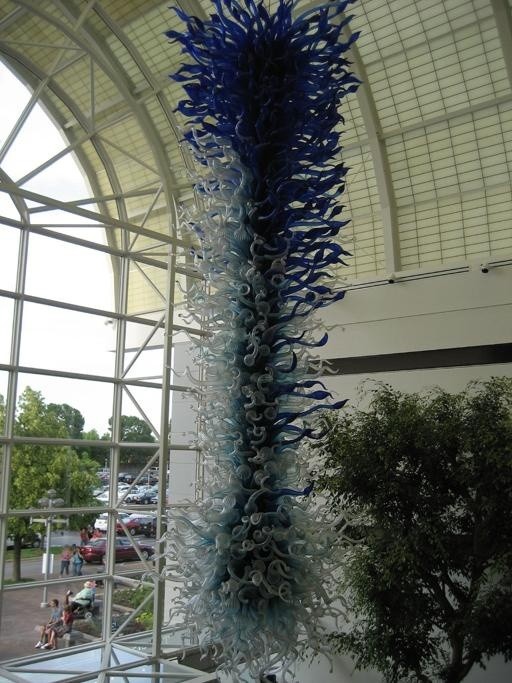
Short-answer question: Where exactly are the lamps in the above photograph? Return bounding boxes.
[479,259,490,273]
[385,271,397,283]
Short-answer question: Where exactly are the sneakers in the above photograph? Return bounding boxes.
[35,643,42,648]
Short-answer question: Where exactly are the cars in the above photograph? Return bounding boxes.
[81,536,155,564]
[90,465,170,503]
[6,529,41,550]
[92,511,166,538]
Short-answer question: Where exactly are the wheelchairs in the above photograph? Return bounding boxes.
[69,591,95,620]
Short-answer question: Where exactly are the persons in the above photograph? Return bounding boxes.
[94,528,102,538]
[40,606,73,650]
[87,527,94,538]
[72,547,83,576]
[70,581,94,612]
[59,544,71,578]
[80,527,89,545]
[35,599,63,648]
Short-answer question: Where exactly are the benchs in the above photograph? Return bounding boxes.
[33,621,84,650]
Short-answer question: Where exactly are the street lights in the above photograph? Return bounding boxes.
[28,489,70,608]
[105,458,108,477]
